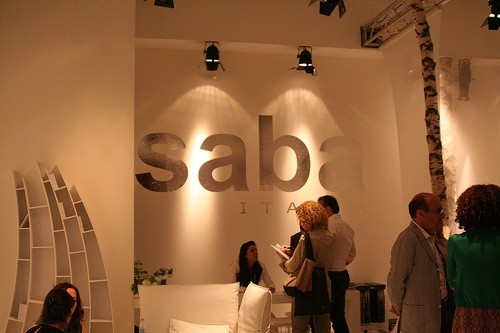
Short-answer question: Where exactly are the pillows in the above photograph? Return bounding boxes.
[238,281,272,333]
[138,282,240,333]
[170,318,229,333]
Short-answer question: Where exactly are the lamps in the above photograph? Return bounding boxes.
[480,0,500,30]
[194,41,225,72]
[288,46,317,76]
[308,0,346,18]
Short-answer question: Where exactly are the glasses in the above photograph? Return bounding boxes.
[422,208,444,214]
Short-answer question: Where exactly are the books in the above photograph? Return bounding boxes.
[271,242,291,260]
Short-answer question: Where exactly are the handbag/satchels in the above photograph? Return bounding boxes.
[283,232,317,297]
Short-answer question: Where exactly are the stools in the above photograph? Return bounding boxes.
[270,317,292,333]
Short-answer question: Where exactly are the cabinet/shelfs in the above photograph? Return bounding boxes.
[2,161,114,333]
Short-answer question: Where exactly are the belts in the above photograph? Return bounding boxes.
[441,296,450,308]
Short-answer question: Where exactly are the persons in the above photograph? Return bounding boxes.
[228,239,278,319]
[316,194,357,332]
[273,200,337,332]
[53,282,84,332]
[387,192,455,333]
[437,219,449,250]
[25,287,75,333]
[446,183,500,332]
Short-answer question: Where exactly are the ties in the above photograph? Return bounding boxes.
[434,240,453,309]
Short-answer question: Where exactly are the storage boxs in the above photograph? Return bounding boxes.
[354,282,387,326]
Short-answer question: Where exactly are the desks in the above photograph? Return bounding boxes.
[238,286,360,333]
